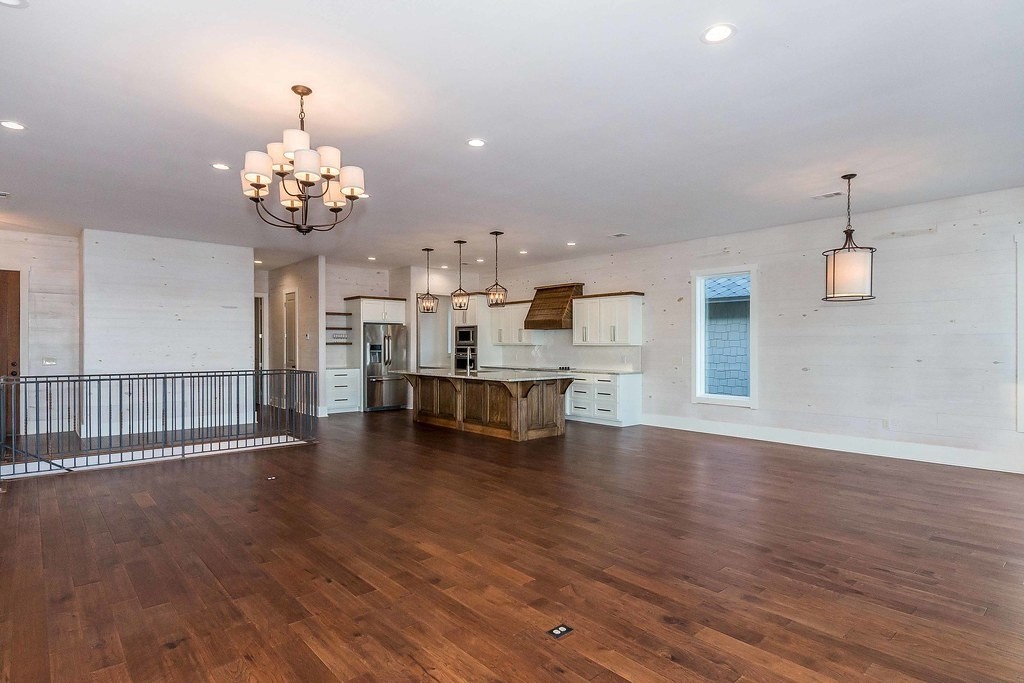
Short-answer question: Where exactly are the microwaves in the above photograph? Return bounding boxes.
[453,325,477,345]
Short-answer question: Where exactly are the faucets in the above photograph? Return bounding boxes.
[465,346,472,377]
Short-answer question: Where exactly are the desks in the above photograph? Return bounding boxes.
[390,365,575,444]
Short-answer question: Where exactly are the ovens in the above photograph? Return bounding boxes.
[455,346,477,378]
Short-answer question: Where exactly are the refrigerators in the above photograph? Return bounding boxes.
[363,322,406,412]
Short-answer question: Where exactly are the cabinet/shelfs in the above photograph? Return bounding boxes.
[325,369,362,413]
[571,368,640,423]
[570,292,644,346]
[491,303,545,346]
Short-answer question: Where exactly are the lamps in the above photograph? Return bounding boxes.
[240,84,368,236]
[820,172,879,304]
[418,248,439,315]
[450,238,471,312]
[486,231,508,309]
